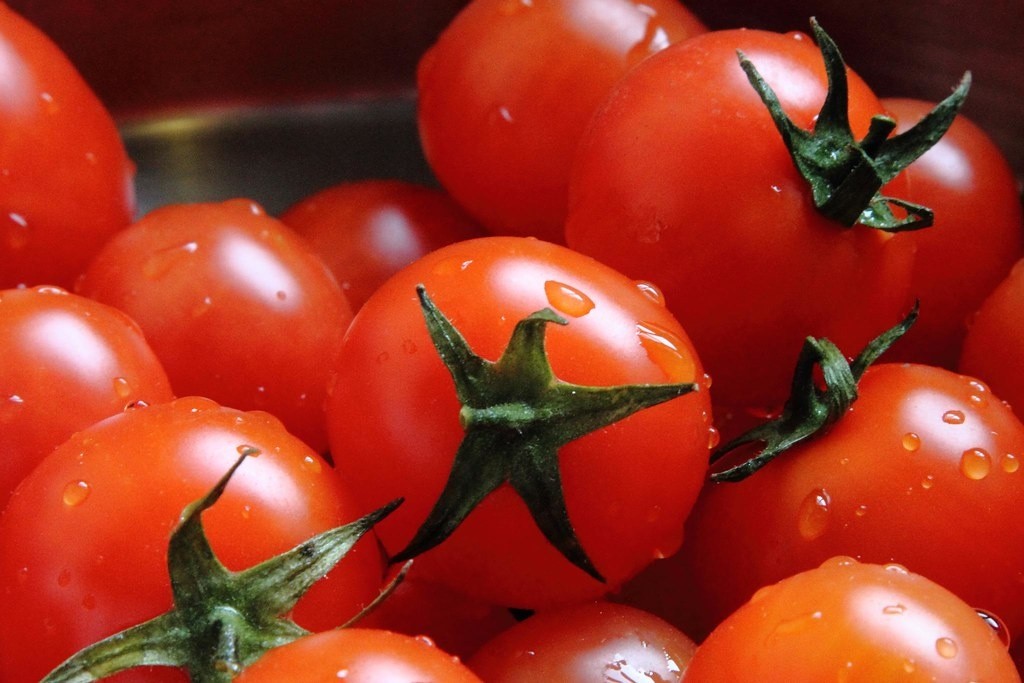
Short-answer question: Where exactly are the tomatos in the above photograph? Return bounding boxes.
[0,0,1024,682]
